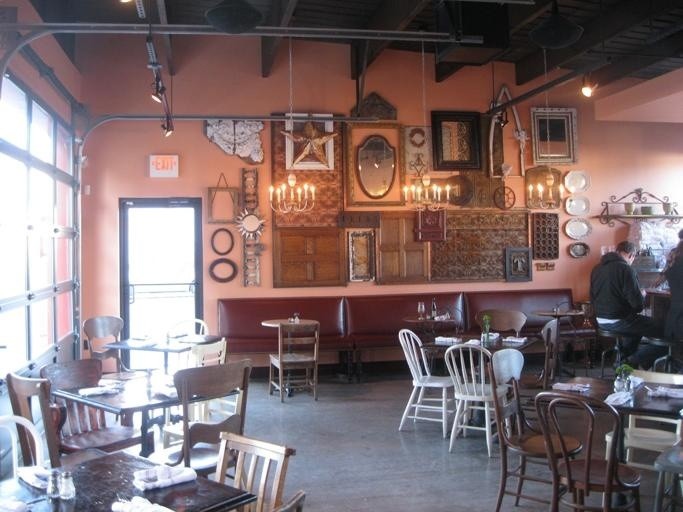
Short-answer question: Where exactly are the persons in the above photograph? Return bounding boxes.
[650,229,683,289]
[589,241,669,371]
[651,241,683,374]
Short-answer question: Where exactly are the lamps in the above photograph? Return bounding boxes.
[152,69,167,104]
[161,104,175,138]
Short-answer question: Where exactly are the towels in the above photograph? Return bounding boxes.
[464,338,481,346]
[628,374,645,389]
[603,391,633,406]
[97,378,125,389]
[434,335,462,343]
[482,332,500,340]
[552,382,591,393]
[130,464,198,491]
[1,496,27,512]
[130,334,147,342]
[15,465,52,490]
[154,382,180,399]
[109,496,177,512]
[656,386,682,399]
[501,335,528,344]
[77,386,118,398]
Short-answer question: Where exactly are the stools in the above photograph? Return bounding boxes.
[640,335,683,373]
[597,330,636,379]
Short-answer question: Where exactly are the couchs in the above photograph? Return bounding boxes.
[209,287,595,377]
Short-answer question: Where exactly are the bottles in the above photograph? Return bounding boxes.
[59,472,76,500]
[432,297,437,320]
[46,471,60,499]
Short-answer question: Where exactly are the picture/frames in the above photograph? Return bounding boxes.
[285,111,334,171]
[530,106,580,167]
[430,110,483,171]
[345,119,405,209]
[504,245,533,283]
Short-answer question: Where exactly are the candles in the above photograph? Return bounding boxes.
[437,187,441,201]
[269,186,274,204]
[304,184,309,201]
[276,188,281,206]
[540,187,544,200]
[537,184,540,197]
[403,187,408,203]
[445,183,451,200]
[411,185,415,199]
[558,185,565,202]
[528,184,531,199]
[433,185,438,200]
[297,189,302,202]
[311,186,316,202]
[280,184,286,201]
[416,187,422,201]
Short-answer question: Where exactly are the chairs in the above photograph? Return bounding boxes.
[605,369,683,512]
[490,376,584,512]
[534,389,645,511]
[190,338,229,368]
[146,358,253,477]
[520,319,559,435]
[2,415,45,477]
[475,307,528,349]
[82,315,150,382]
[3,371,105,466]
[216,431,307,510]
[38,358,155,459]
[444,343,512,456]
[398,329,454,439]
[475,308,527,338]
[194,319,206,336]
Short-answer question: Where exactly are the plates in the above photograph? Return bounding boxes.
[565,218,592,240]
[565,170,591,194]
[570,242,590,258]
[565,195,591,217]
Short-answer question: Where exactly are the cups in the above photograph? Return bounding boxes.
[417,302,425,321]
